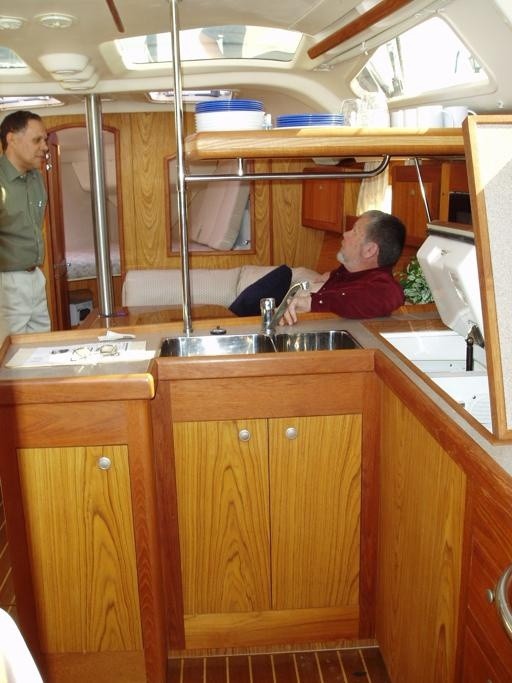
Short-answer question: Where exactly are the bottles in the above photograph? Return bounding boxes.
[343,92,390,130]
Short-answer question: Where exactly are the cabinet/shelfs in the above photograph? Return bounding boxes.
[391,163,449,247]
[373,352,512,683]
[150,373,376,653]
[3,393,169,683]
[301,164,364,235]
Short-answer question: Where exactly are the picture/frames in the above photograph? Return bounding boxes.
[164,155,256,256]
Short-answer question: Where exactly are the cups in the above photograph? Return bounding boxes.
[392,107,477,127]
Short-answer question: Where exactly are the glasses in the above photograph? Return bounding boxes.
[70,344,118,362]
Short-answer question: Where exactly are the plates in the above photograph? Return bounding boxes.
[193,102,346,131]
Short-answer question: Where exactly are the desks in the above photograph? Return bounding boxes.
[79,302,235,330]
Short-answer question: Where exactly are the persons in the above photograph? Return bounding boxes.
[0,110,51,347]
[274,210,405,326]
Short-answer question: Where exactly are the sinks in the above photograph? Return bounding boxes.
[273,332,362,349]
[157,335,274,354]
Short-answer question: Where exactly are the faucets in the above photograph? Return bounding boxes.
[258,280,310,330]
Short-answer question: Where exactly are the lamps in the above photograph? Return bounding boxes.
[50,67,94,81]
[59,74,99,90]
[38,53,89,74]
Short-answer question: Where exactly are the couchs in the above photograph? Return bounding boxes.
[121,264,326,315]
